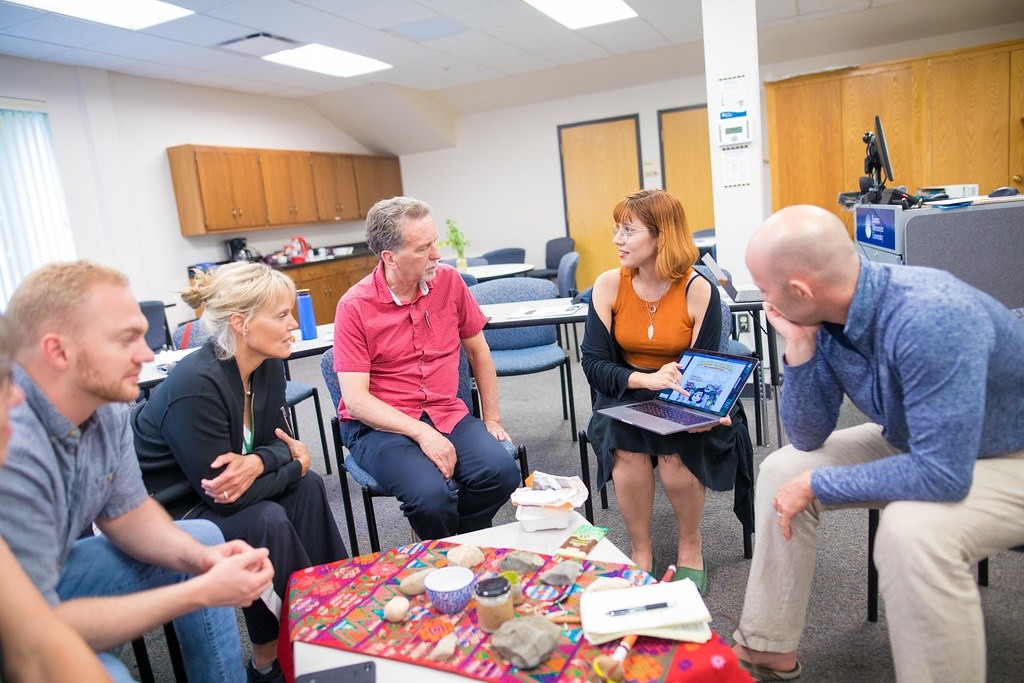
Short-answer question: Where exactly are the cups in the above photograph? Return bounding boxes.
[317,248,328,258]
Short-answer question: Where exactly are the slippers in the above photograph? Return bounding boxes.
[644,556,656,578]
[672,562,707,596]
[732,647,801,679]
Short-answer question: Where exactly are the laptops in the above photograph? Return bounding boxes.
[701,252,765,302]
[597,346,759,436]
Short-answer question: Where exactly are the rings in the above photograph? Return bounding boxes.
[223,491,229,500]
[674,379,677,384]
[777,512,782,517]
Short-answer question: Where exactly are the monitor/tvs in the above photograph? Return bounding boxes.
[864,115,894,203]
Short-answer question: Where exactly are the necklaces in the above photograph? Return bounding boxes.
[244,381,252,396]
[637,273,668,340]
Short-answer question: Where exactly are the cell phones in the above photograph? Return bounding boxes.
[296,662,376,683]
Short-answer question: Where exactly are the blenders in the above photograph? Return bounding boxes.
[225,237,253,262]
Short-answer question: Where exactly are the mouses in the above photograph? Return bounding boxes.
[930,193,949,200]
[988,187,1017,197]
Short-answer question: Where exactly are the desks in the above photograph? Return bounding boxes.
[480,296,589,330]
[466,264,533,280]
[138,322,336,388]
[276,512,753,683]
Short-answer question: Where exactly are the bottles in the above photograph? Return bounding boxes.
[296,293,318,339]
[500,570,524,604]
[474,577,514,634]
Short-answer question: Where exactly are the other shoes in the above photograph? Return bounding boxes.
[247,657,286,683]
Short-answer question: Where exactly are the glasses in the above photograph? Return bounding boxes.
[610,225,655,241]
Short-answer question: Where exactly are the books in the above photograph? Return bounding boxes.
[580,577,713,644]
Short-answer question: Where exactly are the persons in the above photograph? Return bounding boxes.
[130,261,352,683]
[580,189,732,597]
[333,196,521,542]
[0,258,274,683]
[731,204,1023,682]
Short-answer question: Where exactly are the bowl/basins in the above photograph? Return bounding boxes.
[333,247,354,256]
[424,566,474,614]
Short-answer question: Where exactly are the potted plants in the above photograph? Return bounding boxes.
[437,218,470,271]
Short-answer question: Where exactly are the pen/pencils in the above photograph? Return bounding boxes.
[425,310,430,327]
[608,602,668,616]
[553,616,580,623]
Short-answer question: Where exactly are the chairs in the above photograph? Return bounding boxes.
[137,301,168,352]
[468,277,578,442]
[321,342,529,558]
[173,317,333,475]
[483,248,526,264]
[580,301,753,559]
[526,237,575,279]
[556,251,582,363]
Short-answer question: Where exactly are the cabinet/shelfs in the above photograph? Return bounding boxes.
[167,145,404,237]
[283,254,381,328]
